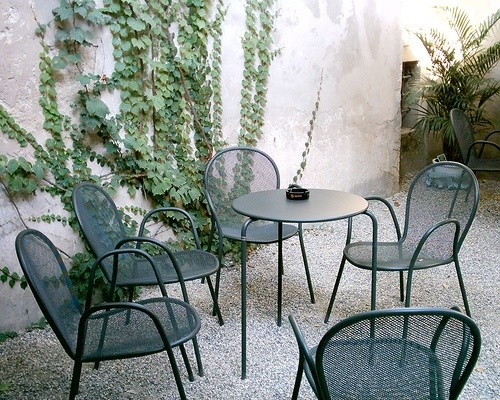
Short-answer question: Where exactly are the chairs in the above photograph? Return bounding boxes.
[201,146,315,316]
[288,305,481,400]
[72,183,224,377]
[447,108,500,219]
[324,161,480,336]
[15,229,195,400]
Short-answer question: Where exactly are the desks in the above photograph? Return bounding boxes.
[232,189,378,380]
